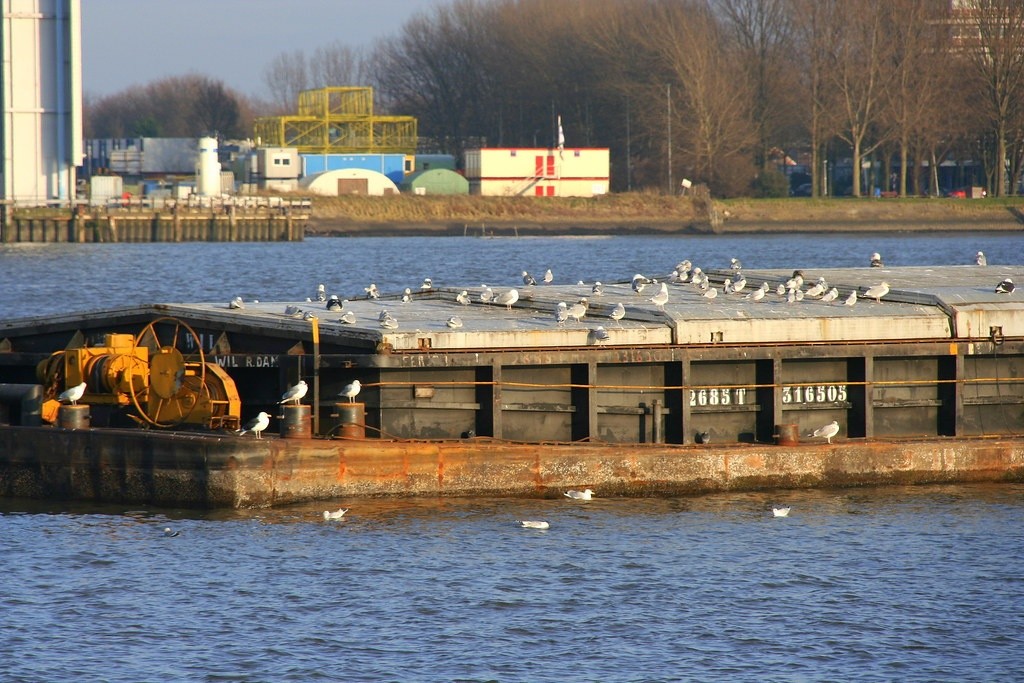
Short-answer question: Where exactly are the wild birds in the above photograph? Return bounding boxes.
[339,278,519,329]
[976,252,986,265]
[254,284,343,311]
[276,381,308,405]
[995,279,1015,293]
[862,282,890,303]
[870,253,883,268]
[240,412,272,439]
[515,489,790,529]
[337,380,362,404]
[521,258,857,348]
[165,509,348,538]
[230,297,244,309]
[808,421,839,444]
[285,305,318,322]
[58,381,88,406]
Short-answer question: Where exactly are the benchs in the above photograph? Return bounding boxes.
[881,192,899,198]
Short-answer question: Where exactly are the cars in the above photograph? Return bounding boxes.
[777,164,985,198]
